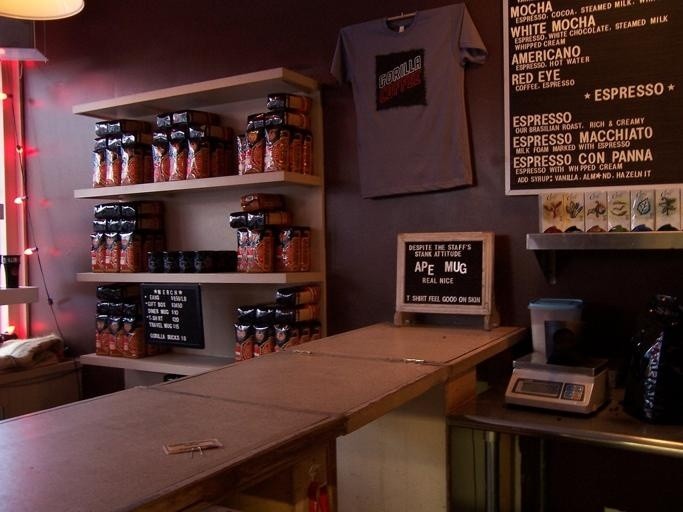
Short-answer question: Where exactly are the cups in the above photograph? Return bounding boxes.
[2,254,20,288]
[543,319,566,364]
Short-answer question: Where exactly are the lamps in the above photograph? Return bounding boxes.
[0,0,84,21]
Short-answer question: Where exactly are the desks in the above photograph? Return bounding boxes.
[465,360,683,509]
[0,323,531,510]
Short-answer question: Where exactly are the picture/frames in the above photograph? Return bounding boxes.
[393,231,499,333]
[499,0,682,197]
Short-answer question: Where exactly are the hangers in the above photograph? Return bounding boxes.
[385,7,416,33]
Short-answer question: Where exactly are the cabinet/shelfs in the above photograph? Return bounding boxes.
[72,67,328,392]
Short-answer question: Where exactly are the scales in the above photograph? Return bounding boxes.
[504,352,610,415]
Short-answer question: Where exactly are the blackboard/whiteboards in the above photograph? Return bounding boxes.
[395,232,495,316]
[140,282,206,349]
